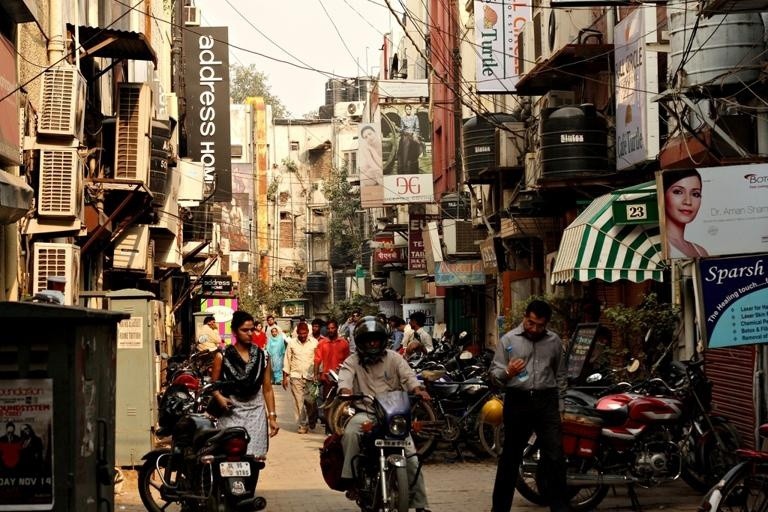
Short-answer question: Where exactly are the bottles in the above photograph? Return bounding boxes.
[506,345,531,384]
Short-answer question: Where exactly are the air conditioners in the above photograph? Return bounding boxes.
[441,218,490,257]
[280,301,305,318]
[27,64,219,311]
[345,101,367,118]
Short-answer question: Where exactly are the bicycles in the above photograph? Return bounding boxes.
[646,319,691,379]
[697,422,768,511]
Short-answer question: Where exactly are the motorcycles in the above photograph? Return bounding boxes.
[167,334,223,412]
[514,354,645,508]
[138,378,267,512]
[315,330,504,460]
[333,391,434,511]
[534,338,751,512]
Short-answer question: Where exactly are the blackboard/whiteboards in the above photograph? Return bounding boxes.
[567,323,601,378]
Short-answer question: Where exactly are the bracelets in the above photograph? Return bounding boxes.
[269,412,277,418]
[505,369,511,377]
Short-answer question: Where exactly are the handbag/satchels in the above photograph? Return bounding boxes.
[319,432,346,492]
[206,348,232,419]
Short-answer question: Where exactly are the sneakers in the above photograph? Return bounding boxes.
[297,424,308,433]
[309,416,316,429]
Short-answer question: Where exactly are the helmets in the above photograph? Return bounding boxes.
[353,315,389,357]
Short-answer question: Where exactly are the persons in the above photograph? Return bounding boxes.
[359,126,382,168]
[663,170,710,257]
[488,301,567,511]
[334,314,433,512]
[227,196,249,251]
[206,309,280,500]
[19,424,43,476]
[197,316,222,354]
[252,310,433,437]
[392,104,420,144]
[0,422,20,477]
[232,166,246,194]
